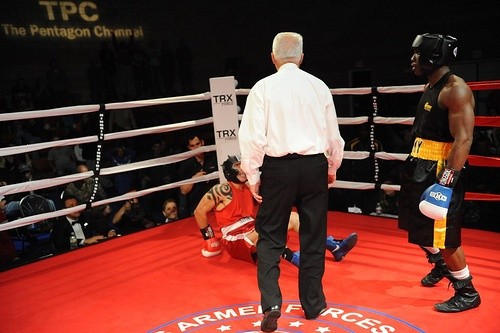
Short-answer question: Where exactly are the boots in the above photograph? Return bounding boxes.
[434,274,481,312]
[420,252,447,286]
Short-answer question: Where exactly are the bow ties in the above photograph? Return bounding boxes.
[72,220,80,225]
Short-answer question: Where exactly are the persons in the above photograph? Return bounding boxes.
[397,34,481,313]
[236,32,345,332]
[329,89,500,231]
[0,30,218,270]
[194,155,358,269]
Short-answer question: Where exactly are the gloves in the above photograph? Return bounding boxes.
[200,224,222,257]
[418,166,460,221]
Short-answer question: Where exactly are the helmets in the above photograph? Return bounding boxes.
[413,32,459,74]
[221,154,245,184]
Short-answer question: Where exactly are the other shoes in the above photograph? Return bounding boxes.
[261,305,281,332]
[305,301,327,319]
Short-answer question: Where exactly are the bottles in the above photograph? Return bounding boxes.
[375,202,382,217]
[69,232,78,250]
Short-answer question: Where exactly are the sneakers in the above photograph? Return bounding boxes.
[326,233,357,261]
[292,250,300,266]
